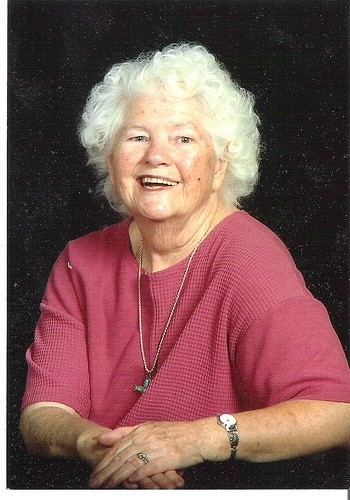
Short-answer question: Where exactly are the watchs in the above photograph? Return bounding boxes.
[216,413,241,462]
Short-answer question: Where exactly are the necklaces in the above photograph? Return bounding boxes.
[133,208,227,392]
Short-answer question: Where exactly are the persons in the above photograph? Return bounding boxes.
[19,41,349,488]
